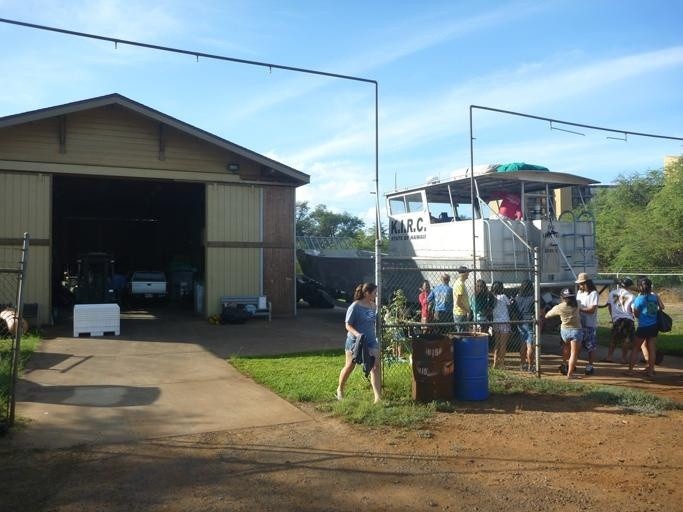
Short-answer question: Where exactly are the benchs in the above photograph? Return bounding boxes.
[220,295,272,322]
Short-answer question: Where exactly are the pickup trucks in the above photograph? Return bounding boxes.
[127,270,170,306]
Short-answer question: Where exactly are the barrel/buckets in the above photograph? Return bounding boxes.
[412,333,454,401]
[0,307,30,334]
[449,333,489,400]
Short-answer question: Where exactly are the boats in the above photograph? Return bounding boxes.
[293,165,602,333]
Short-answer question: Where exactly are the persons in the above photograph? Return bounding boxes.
[384,294,409,362]
[470,279,497,367]
[512,280,546,374]
[418,280,432,334]
[453,266,472,332]
[491,281,513,370]
[575,273,599,375]
[336,283,383,403]
[544,288,584,379]
[600,278,665,377]
[426,273,456,335]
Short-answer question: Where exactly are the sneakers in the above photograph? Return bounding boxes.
[656,349,663,364]
[585,364,594,373]
[558,362,576,374]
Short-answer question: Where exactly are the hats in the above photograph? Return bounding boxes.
[561,288,575,298]
[575,273,591,284]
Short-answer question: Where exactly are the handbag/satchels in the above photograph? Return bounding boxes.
[658,310,672,333]
